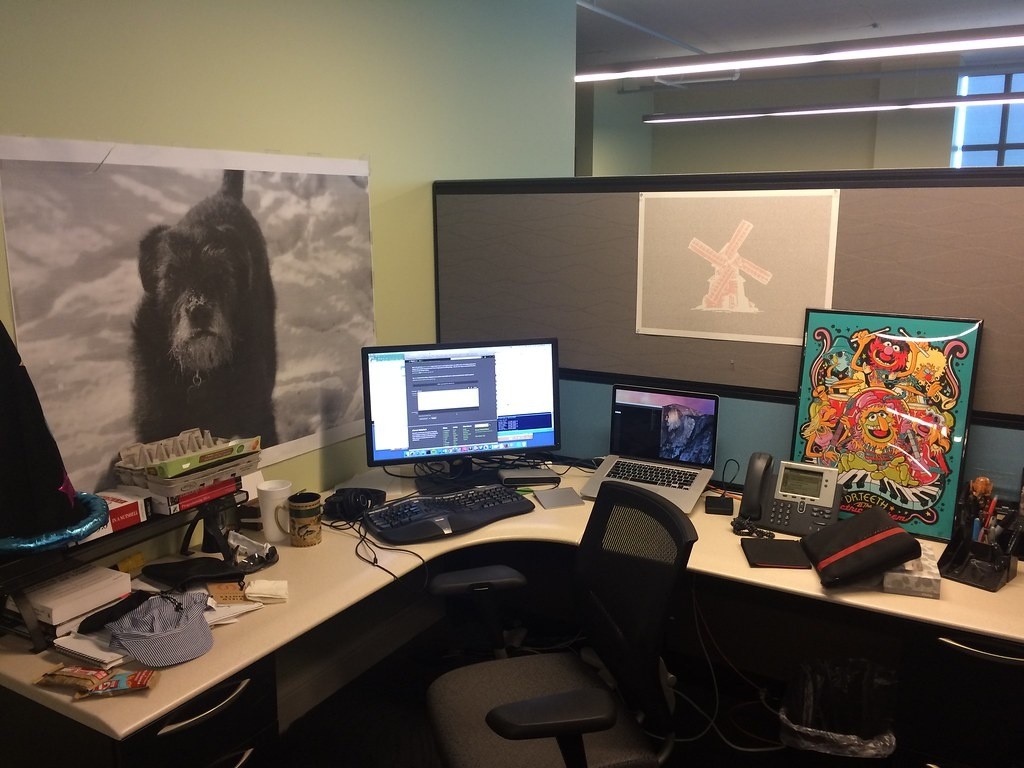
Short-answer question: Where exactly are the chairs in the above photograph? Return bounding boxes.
[424,480,697,768]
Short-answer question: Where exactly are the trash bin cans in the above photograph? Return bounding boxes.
[773,660,900,768]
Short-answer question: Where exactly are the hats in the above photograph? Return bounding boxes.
[102,589,214,666]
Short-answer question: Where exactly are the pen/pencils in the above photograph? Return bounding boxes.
[972,494,1023,550]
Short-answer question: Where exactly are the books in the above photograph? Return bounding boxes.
[42,487,153,548]
[3,565,265,670]
[116,475,242,514]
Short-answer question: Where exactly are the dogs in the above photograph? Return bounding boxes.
[125,168,281,452]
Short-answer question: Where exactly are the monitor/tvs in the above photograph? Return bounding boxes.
[361,337,561,496]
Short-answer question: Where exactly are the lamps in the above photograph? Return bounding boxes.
[575,24,1024,86]
[642,91,1024,124]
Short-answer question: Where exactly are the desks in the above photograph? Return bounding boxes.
[0,453,1024,768]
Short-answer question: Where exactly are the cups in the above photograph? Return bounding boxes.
[274,492,323,547]
[255,480,293,542]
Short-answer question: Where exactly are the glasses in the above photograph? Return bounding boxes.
[235,546,279,574]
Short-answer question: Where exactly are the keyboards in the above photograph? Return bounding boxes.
[362,485,535,545]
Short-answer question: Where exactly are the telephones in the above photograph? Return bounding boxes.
[735,451,846,537]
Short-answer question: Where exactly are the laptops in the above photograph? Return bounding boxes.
[580,384,720,514]
